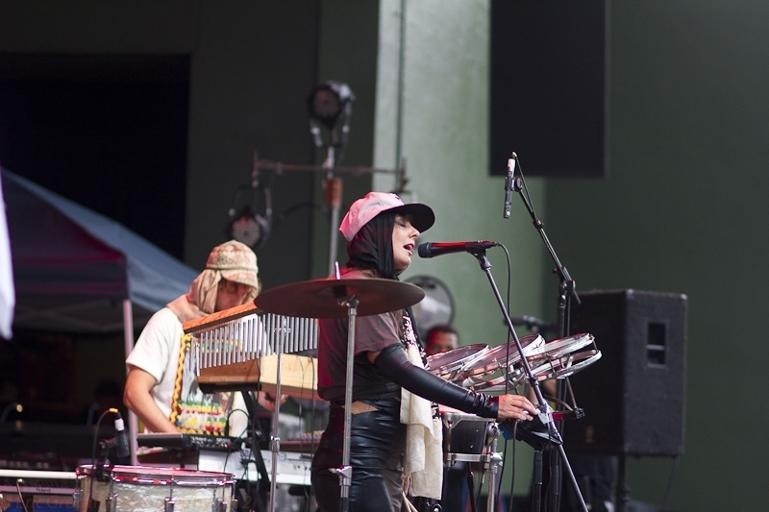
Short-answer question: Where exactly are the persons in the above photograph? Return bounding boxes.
[310,192,537,511]
[86,379,126,419]
[403,322,496,510]
[126,235,287,461]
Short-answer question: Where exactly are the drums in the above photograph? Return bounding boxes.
[462,335,544,390]
[525,333,602,381]
[75,465,238,512]
[442,411,497,462]
[425,343,498,382]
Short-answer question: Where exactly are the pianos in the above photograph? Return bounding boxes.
[101,431,323,486]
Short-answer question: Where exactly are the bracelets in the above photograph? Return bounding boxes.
[263,392,284,404]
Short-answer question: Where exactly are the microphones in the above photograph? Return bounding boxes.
[417,238,498,258]
[503,158,516,218]
[112,412,131,465]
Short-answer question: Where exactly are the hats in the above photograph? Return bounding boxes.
[203,238,261,292]
[338,190,436,243]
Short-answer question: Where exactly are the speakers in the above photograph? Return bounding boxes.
[562,288,691,458]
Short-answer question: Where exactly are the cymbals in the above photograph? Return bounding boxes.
[253,279,425,319]
